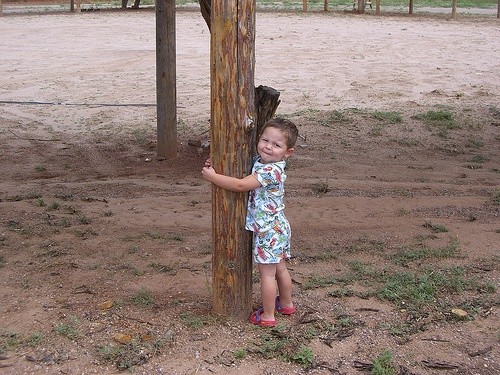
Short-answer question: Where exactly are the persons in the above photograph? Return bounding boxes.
[201,116,295,328]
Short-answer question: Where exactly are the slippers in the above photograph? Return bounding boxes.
[249,307,275,327]
[275,295,295,315]
